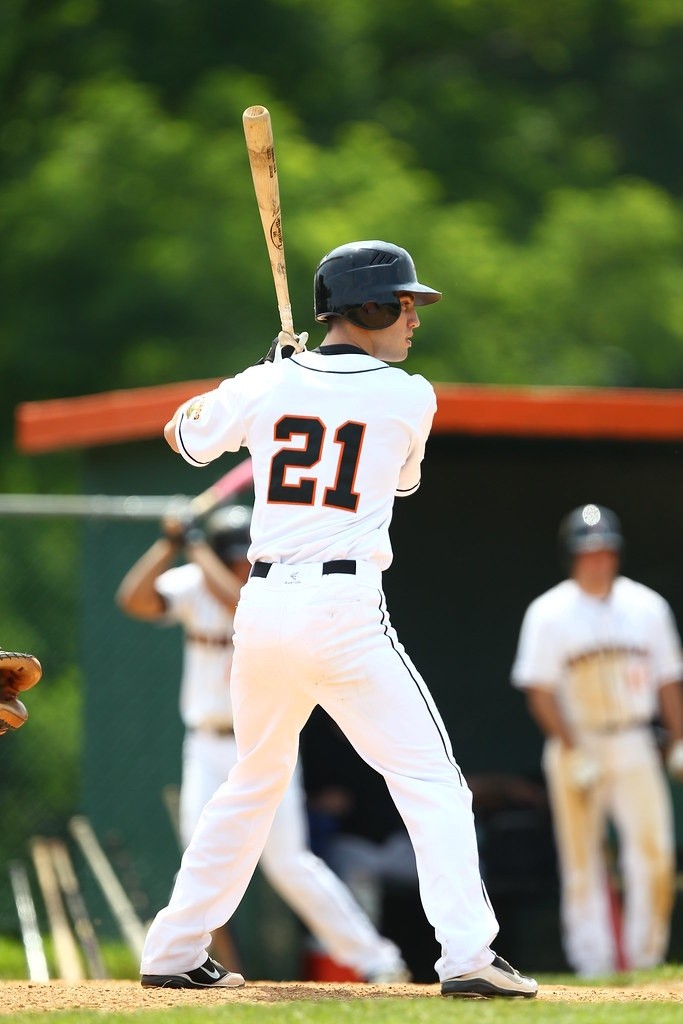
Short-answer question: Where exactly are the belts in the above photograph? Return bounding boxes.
[183,725,236,739]
[599,716,653,737]
[250,560,358,579]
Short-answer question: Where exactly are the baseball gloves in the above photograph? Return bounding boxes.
[0,645,43,735]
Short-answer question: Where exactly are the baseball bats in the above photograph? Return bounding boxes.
[11,810,149,980]
[176,458,252,519]
[241,104,297,342]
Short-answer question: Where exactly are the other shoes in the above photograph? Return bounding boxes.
[575,965,621,983]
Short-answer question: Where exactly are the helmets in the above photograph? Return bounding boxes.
[558,502,625,567]
[202,504,254,567]
[313,239,443,331]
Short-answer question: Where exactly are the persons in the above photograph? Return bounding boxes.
[118,504,413,984]
[140,240,538,1000]
[510,505,683,975]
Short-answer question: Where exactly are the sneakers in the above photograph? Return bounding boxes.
[440,953,540,1003]
[367,969,411,985]
[140,953,246,990]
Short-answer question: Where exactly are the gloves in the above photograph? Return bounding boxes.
[561,736,598,802]
[253,330,309,365]
[665,737,683,784]
[162,496,208,546]
